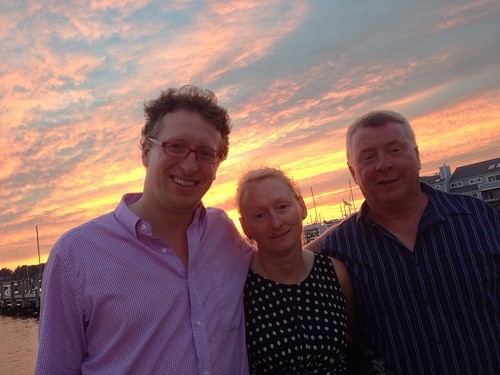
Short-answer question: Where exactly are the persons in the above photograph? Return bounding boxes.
[233,167,372,375]
[319,112,500,375]
[34,84,340,375]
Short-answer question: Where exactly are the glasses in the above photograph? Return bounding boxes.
[147,135,223,164]
[245,202,300,225]
